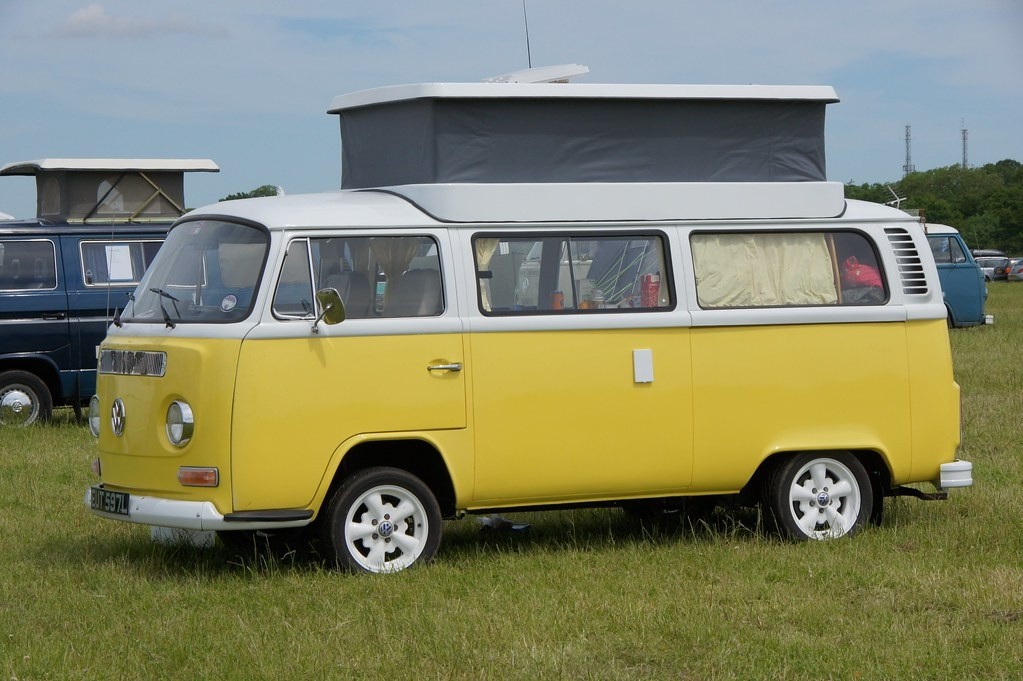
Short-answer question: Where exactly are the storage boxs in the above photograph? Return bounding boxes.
[985,315,993,324]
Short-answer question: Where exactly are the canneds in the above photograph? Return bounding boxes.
[551,290,564,309]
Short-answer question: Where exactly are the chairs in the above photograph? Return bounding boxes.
[382,268,442,316]
[341,269,372,318]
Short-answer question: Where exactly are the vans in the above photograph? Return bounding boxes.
[586,222,992,329]
[0,157,330,434]
[79,79,977,575]
[971,257,1010,283]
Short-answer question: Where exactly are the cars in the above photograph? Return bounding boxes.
[1006,258,1023,281]
[993,257,1022,280]
